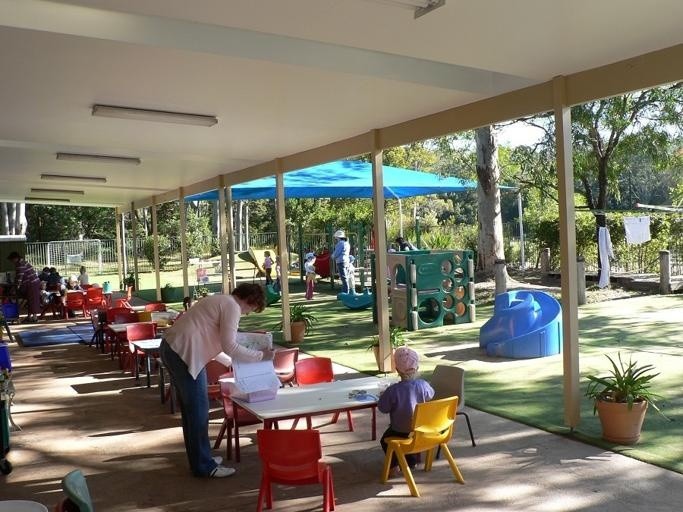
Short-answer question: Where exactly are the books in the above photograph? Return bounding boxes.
[233,331,281,395]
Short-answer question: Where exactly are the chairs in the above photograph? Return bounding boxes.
[1,281,111,320]
[429,366,476,461]
[290,356,355,435]
[213,372,279,463]
[256,429,335,512]
[90,298,302,420]
[381,396,465,497]
[1,320,96,510]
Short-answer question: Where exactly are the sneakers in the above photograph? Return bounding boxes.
[210,456,224,465]
[200,466,237,479]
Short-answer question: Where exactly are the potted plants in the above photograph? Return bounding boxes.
[583,351,667,445]
[367,327,410,372]
[272,303,318,343]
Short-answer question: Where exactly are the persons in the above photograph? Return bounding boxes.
[169,295,192,325]
[330,230,350,300]
[303,252,317,300]
[261,250,273,285]
[347,255,356,294]
[6,251,41,324]
[394,236,419,251]
[274,255,282,292]
[196,264,208,284]
[37,266,87,317]
[385,249,395,279]
[376,346,434,476]
[158,283,277,477]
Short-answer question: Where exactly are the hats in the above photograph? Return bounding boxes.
[333,230,345,238]
[395,348,419,374]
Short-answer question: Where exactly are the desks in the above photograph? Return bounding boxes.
[229,377,392,462]
